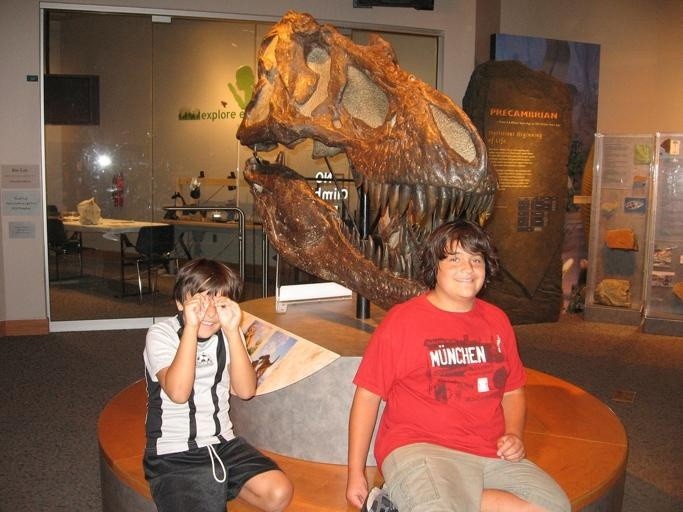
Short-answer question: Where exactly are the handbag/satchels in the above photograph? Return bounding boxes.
[606,229,638,251]
[593,278,631,307]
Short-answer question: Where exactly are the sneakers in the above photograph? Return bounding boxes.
[360,481,399,512]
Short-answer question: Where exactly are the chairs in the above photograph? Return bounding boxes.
[47,218,83,281]
[120,225,175,293]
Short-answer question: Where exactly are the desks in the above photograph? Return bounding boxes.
[63,219,169,293]
[160,219,262,260]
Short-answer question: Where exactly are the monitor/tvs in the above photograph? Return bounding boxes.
[45,74,100,126]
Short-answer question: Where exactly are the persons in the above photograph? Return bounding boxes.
[142,259,293,512]
[346,220,572,512]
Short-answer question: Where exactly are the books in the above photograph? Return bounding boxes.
[181,215,210,222]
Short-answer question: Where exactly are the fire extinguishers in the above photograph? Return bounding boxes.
[112,172,124,208]
